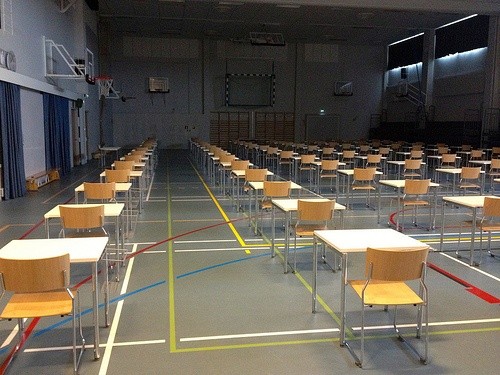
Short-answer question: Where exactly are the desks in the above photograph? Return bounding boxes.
[0,236,110,360]
[100,170,145,217]
[310,161,346,195]
[219,159,253,197]
[395,151,424,159]
[425,147,450,154]
[333,152,358,162]
[353,155,386,169]
[457,150,486,162]
[313,227,430,346]
[112,162,146,169]
[44,203,125,280]
[371,148,393,154]
[291,156,319,184]
[232,169,273,213]
[426,155,461,169]
[270,197,347,273]
[77,182,133,239]
[378,180,440,232]
[387,159,427,180]
[336,169,383,208]
[469,160,493,172]
[434,167,486,196]
[247,181,301,236]
[439,195,500,266]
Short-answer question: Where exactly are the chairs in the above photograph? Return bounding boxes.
[0,137,161,375]
[190,134,500,367]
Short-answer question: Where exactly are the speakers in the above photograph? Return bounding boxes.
[401,68,408,79]
